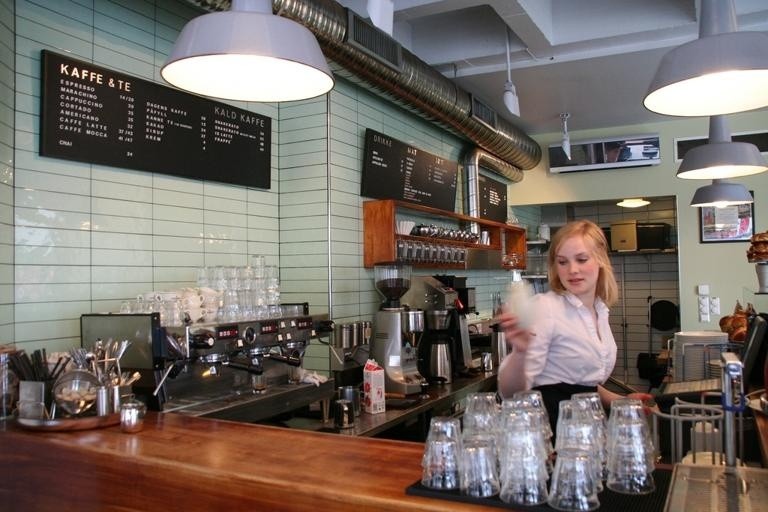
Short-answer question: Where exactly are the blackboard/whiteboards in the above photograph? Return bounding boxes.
[360,127,459,213]
[38,49,272,193]
[478,173,507,224]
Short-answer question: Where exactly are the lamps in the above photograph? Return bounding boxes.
[690,179,754,210]
[559,112,573,159]
[640,0,768,119]
[365,0,395,37]
[501,25,523,118]
[675,113,768,182]
[160,0,334,104]
[615,197,651,208]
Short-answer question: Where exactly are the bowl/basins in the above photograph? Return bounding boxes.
[394,220,415,236]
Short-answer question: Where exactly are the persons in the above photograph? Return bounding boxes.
[497,219,653,448]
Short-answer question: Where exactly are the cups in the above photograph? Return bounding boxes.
[420,389,664,511]
[200,254,282,326]
[334,400,356,429]
[96,386,120,418]
[119,384,133,406]
[118,400,147,434]
[120,287,207,326]
[480,352,493,370]
[502,251,519,267]
[18,380,44,418]
[535,233,541,241]
[338,386,367,417]
[481,231,489,245]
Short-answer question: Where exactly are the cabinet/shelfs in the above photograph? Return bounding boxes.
[363,200,528,270]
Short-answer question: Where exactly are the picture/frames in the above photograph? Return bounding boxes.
[698,190,755,244]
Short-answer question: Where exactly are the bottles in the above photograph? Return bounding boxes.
[334,321,372,347]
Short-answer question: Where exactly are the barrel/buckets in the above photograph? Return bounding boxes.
[650,328,676,355]
[610,219,637,251]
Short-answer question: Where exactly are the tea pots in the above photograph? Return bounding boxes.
[413,224,479,243]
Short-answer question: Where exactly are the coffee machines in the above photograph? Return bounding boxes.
[80,302,337,422]
[400,276,459,384]
[433,274,479,375]
[373,264,429,394]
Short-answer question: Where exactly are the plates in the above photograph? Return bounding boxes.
[672,331,730,382]
[15,413,122,431]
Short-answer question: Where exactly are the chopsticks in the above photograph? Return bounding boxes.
[7,348,72,407]
